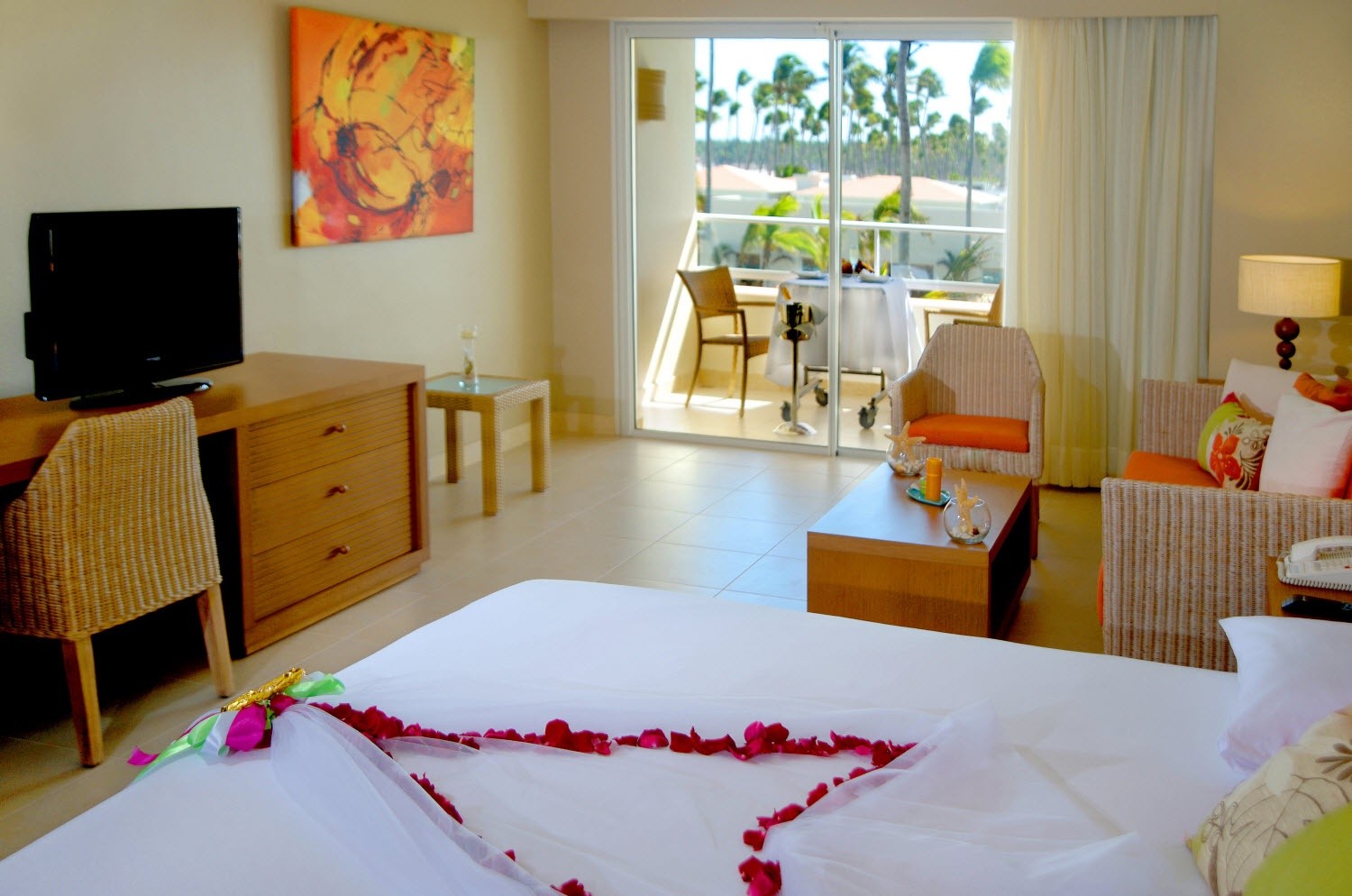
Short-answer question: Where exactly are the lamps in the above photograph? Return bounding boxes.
[1235,255,1342,369]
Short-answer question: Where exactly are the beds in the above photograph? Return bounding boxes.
[0,579,1351,896]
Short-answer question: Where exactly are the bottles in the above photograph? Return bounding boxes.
[943,496,991,545]
[456,323,479,392]
[886,440,925,475]
[925,456,943,501]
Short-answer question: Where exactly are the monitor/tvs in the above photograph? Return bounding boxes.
[24,206,244,410]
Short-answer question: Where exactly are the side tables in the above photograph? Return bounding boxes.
[1262,556,1352,621]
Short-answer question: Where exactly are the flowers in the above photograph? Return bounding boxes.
[461,324,478,378]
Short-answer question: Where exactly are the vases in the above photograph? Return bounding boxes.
[459,339,480,388]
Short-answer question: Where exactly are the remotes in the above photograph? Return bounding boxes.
[1280,593,1352,623]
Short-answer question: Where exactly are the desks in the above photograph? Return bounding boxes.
[425,373,551,515]
[764,274,917,431]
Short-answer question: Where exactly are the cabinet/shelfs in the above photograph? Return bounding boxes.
[0,351,433,654]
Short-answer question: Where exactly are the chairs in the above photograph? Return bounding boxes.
[0,395,233,765]
[676,266,808,418]
[1098,374,1352,673]
[923,280,1003,344]
[890,323,1046,477]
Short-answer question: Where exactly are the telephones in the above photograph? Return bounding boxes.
[1276,534,1352,592]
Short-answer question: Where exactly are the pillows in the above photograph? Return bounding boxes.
[1196,392,1273,490]
[1218,615,1351,777]
[1258,372,1352,499]
[1184,703,1352,895]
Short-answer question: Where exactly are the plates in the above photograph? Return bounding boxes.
[905,484,951,507]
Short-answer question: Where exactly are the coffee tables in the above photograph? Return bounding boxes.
[807,460,1032,639]
